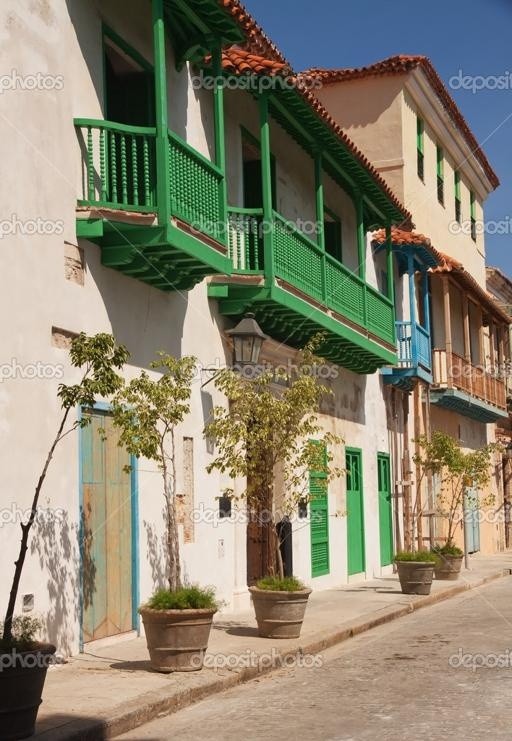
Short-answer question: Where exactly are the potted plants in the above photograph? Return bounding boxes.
[393,426,510,598]
[0,327,352,741]
